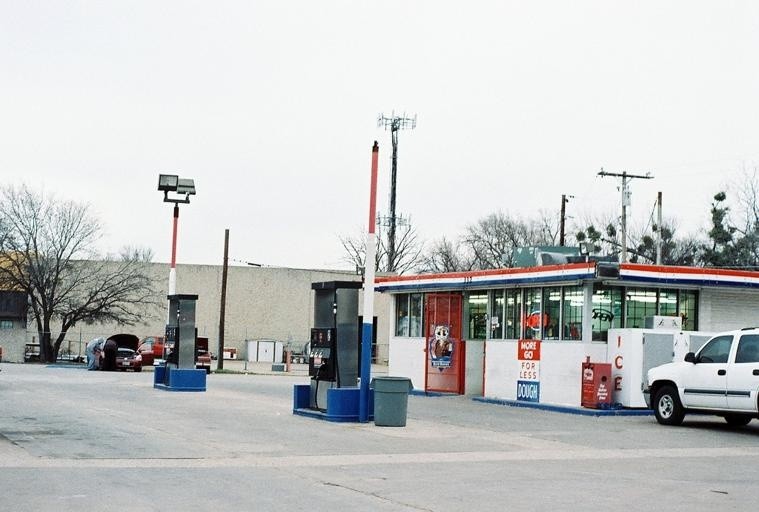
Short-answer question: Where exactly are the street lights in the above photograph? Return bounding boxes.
[157,174,196,365]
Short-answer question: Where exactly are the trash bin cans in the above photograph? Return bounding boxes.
[374,376,410,428]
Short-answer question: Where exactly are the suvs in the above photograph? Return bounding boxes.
[642,326,759,426]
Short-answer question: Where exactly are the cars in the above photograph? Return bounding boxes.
[166,337,212,373]
[138,335,165,360]
[93,333,142,372]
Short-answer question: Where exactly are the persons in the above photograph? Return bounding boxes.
[85,337,104,372]
[101,338,119,371]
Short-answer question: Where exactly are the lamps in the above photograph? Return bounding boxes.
[596,261,619,279]
[580,243,594,263]
